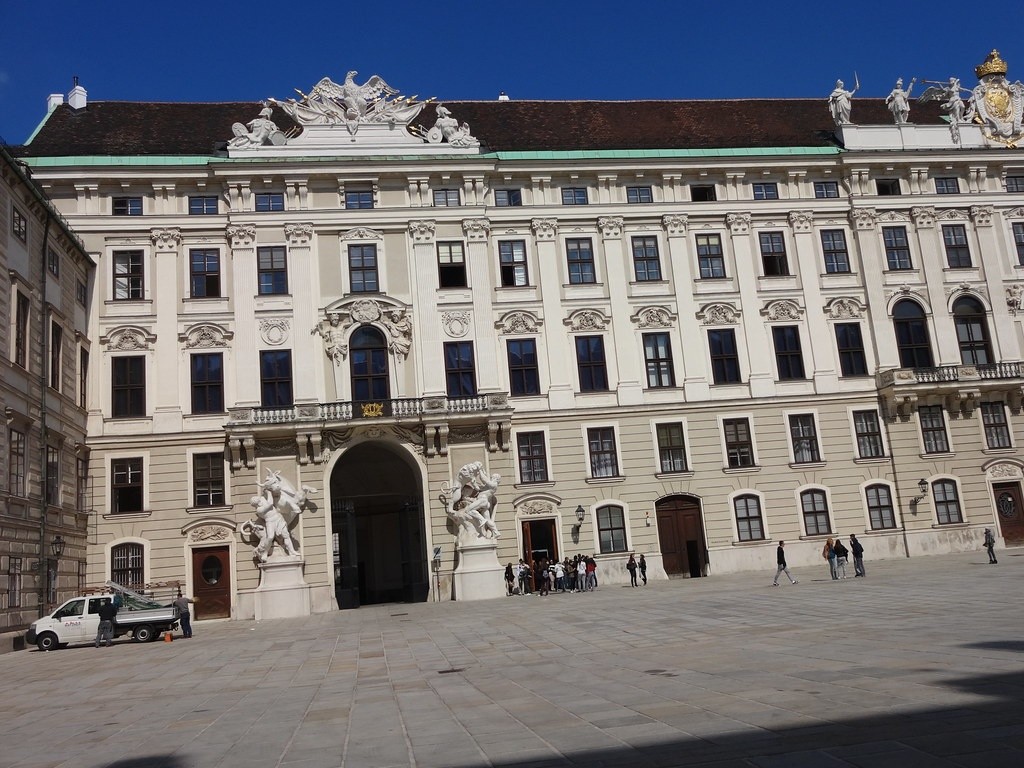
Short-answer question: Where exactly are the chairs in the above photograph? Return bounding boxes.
[89,600,98,614]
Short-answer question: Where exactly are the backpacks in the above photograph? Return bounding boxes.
[578,562,585,574]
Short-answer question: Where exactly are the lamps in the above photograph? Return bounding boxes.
[914,479,929,505]
[574,504,586,531]
[51,536,66,560]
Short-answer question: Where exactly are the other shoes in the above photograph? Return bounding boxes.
[793,581,800,584]
[773,583,779,586]
[538,594,542,596]
[635,585,639,587]
[96,645,99,648]
[992,560,997,563]
[106,643,113,647]
[545,594,548,596]
[989,560,993,564]
[525,592,531,595]
[183,636,191,638]
[632,585,634,588]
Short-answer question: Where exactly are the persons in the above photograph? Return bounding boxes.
[638,555,647,585]
[518,554,597,597]
[628,554,638,587]
[983,528,997,564]
[886,78,916,124]
[850,534,866,577]
[250,489,301,563]
[465,465,501,540]
[828,80,859,126]
[175,593,192,638]
[938,78,965,122]
[378,307,411,355]
[834,539,848,580]
[825,539,838,580]
[773,541,799,586]
[1008,284,1024,315]
[96,598,117,648]
[434,103,476,145]
[322,309,353,355]
[505,563,515,596]
[228,107,277,147]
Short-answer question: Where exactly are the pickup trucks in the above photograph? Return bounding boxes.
[25,592,180,652]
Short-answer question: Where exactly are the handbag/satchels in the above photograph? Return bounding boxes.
[508,580,515,586]
[626,560,631,569]
[542,569,549,579]
[639,574,641,578]
